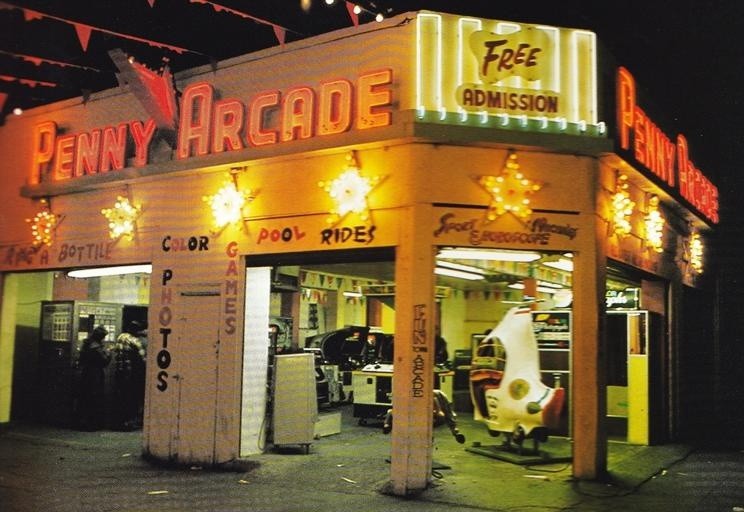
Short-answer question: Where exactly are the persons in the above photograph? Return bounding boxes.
[79,320,148,430]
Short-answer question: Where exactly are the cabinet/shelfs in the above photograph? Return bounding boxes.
[269,346,319,454]
[348,365,456,425]
